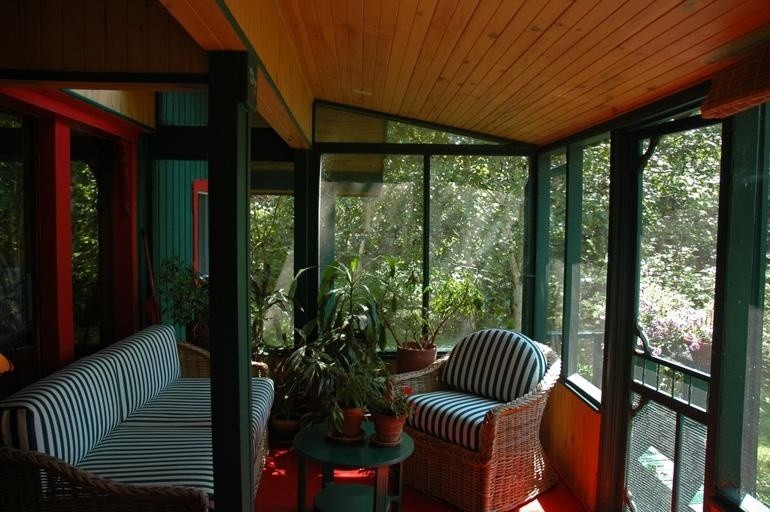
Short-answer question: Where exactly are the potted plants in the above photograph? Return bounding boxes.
[375,262,485,376]
[270,261,419,450]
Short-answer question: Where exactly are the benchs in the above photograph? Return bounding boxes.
[0,323,275,512]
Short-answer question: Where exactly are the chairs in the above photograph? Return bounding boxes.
[376,329,563,511]
